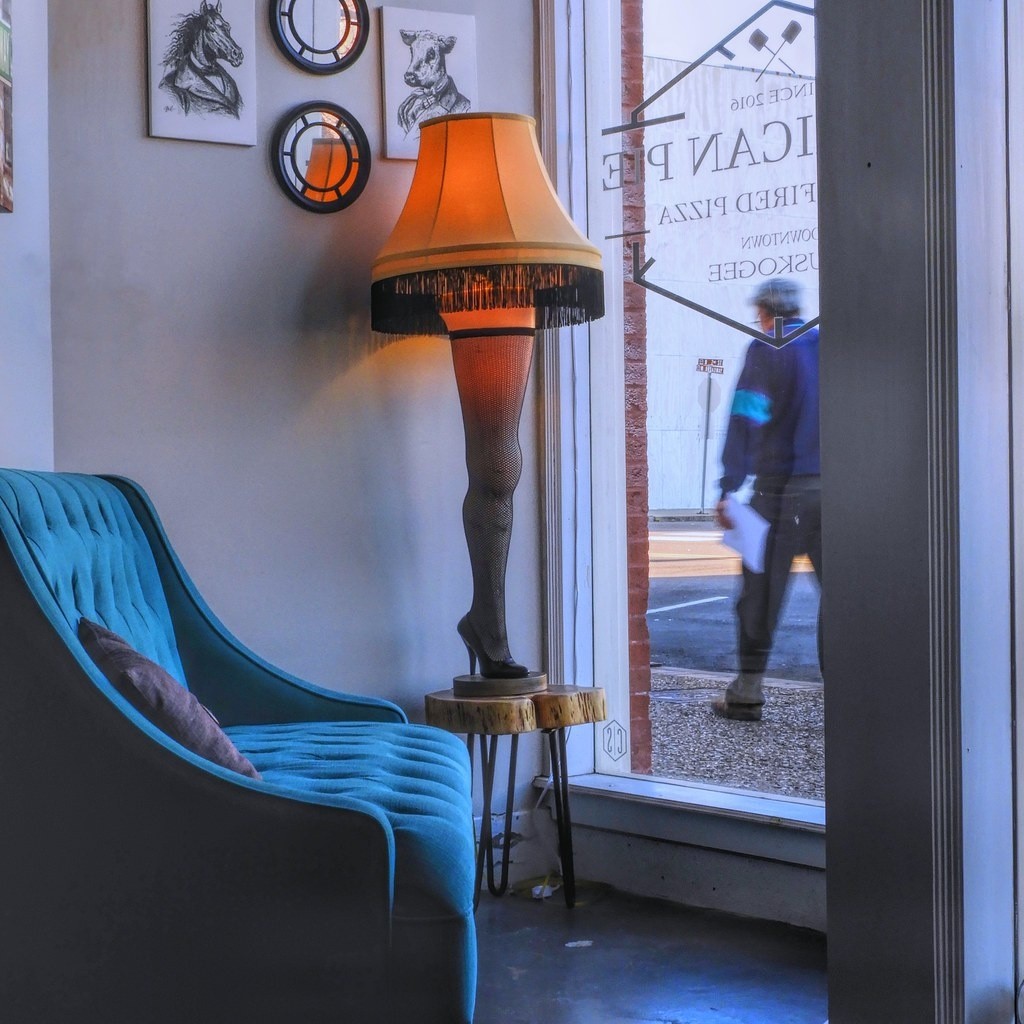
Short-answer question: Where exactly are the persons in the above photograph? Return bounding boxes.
[710,278,824,723]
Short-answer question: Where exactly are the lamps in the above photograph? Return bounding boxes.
[300,134,362,203]
[369,112,603,677]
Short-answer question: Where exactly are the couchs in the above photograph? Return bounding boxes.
[0,465,482,1020]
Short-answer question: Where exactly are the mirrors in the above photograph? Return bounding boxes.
[269,99,374,214]
[268,0,371,76]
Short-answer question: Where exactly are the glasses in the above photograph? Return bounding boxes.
[755,311,764,329]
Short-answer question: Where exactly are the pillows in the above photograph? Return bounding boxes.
[72,614,259,781]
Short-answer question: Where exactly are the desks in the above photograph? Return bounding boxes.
[417,682,607,911]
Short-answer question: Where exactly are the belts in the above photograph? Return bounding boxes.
[752,476,820,491]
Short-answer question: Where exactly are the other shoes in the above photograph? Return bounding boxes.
[711,698,762,721]
